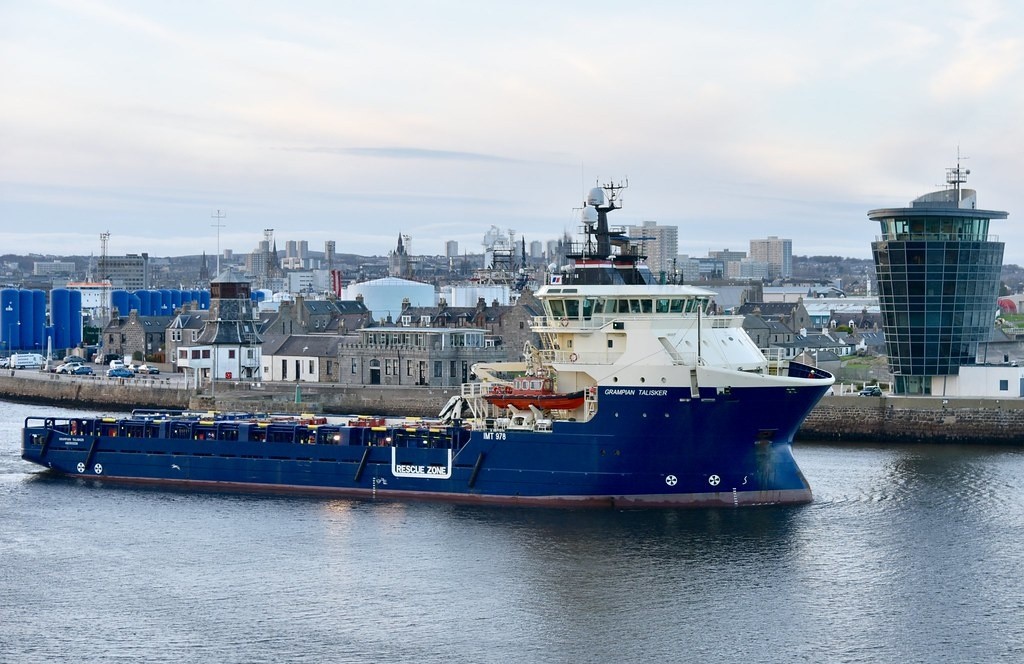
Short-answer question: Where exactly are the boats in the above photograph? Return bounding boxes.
[482,341,596,411]
[21,176,837,510]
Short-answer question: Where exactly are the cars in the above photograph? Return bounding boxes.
[95,355,106,364]
[0,356,10,369]
[56,363,83,374]
[138,365,160,374]
[63,355,86,364]
[857,386,882,397]
[128,365,140,372]
[70,366,93,375]
[109,360,124,369]
[106,367,135,379]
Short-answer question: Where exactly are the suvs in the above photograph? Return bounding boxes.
[100,353,123,364]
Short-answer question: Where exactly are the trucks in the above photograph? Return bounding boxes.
[10,354,47,369]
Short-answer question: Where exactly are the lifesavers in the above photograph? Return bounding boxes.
[492,386,502,397]
[504,385,514,395]
